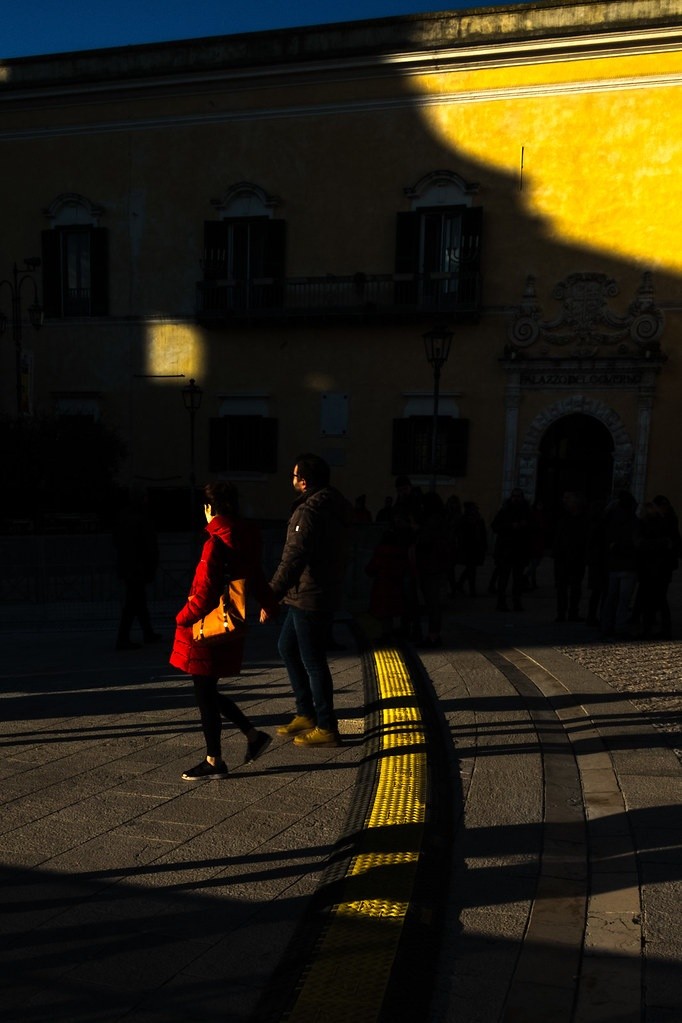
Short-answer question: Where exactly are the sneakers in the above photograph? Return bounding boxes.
[244,730,272,766]
[180,759,229,781]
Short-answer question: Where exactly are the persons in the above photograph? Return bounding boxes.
[164,481,272,783]
[258,459,343,748]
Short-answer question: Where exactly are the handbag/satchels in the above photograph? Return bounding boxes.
[187,578,251,645]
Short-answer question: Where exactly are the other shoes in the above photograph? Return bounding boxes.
[275,717,316,736]
[294,728,344,748]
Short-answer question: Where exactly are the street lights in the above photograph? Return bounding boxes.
[420,318,454,616]
[0,253,45,538]
[183,377,204,598]
